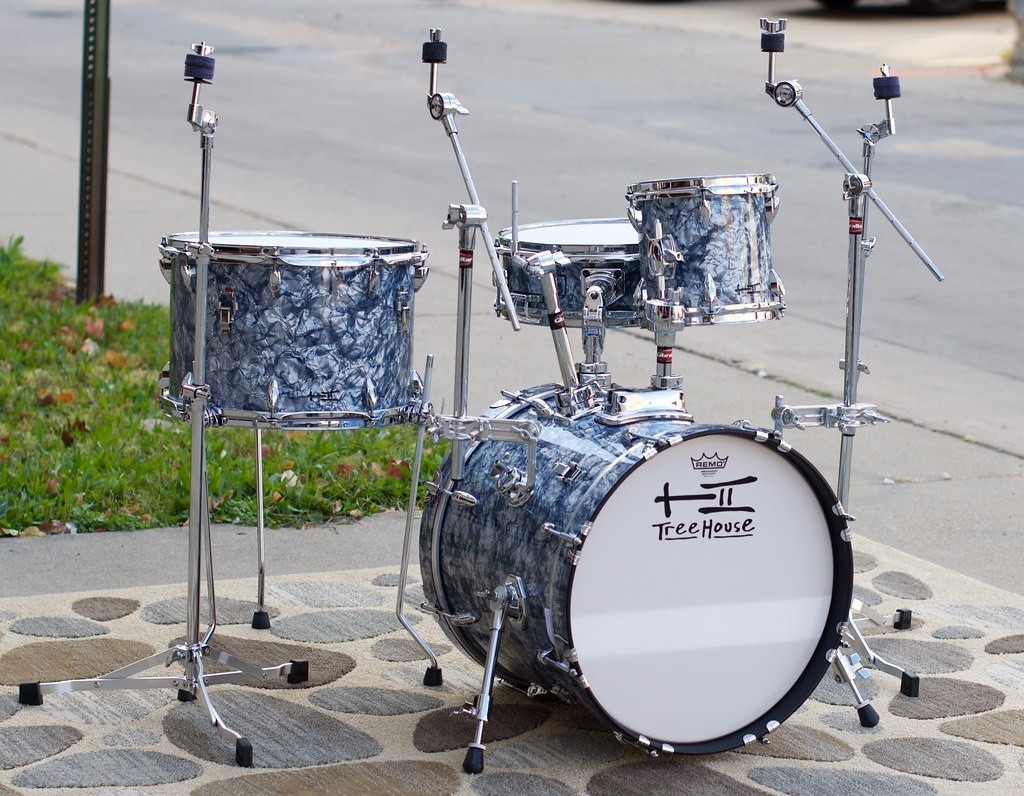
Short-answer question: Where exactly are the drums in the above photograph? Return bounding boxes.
[418,382,855,756]
[489,218,653,329]
[624,170,783,331]
[154,229,426,425]
